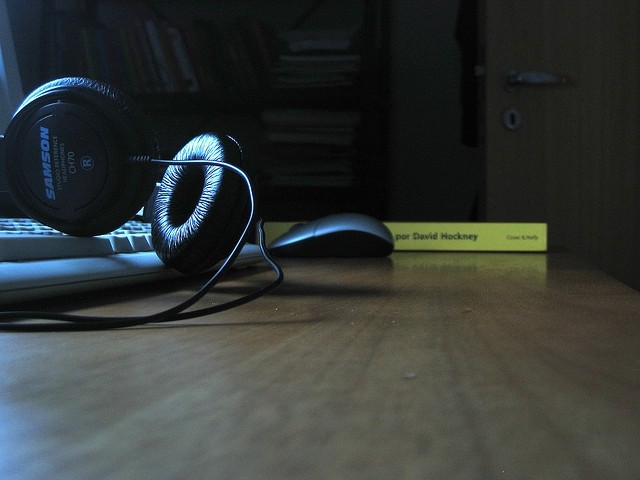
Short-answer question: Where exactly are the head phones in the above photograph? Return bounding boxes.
[2,76,260,274]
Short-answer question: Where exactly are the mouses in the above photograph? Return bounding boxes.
[271,213,390,257]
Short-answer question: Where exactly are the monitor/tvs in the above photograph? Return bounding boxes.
[0,0,41,195]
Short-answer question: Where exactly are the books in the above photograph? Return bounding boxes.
[257,217,548,255]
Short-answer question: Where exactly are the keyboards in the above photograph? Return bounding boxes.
[2,215,268,302]
